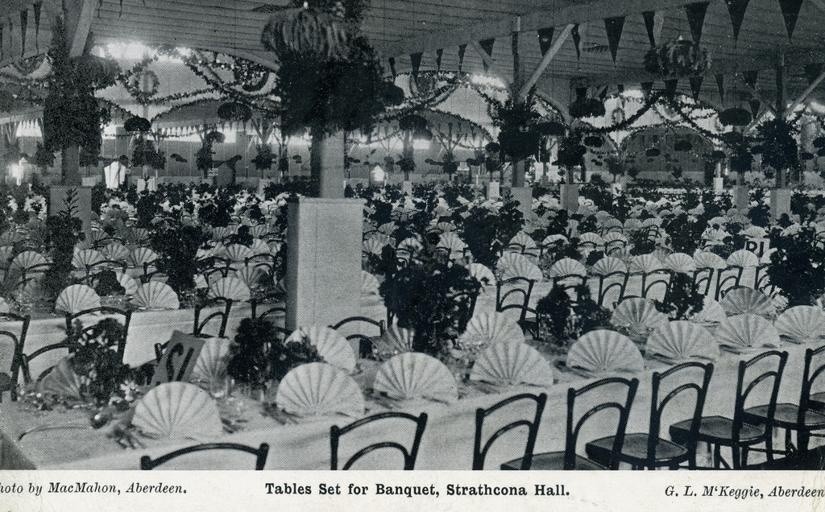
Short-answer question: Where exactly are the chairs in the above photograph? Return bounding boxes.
[0,168,824,470]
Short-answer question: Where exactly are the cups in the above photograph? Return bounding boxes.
[207,371,230,407]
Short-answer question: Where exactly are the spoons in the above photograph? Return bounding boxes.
[15,411,115,444]
[112,424,145,451]
[221,310,802,435]
